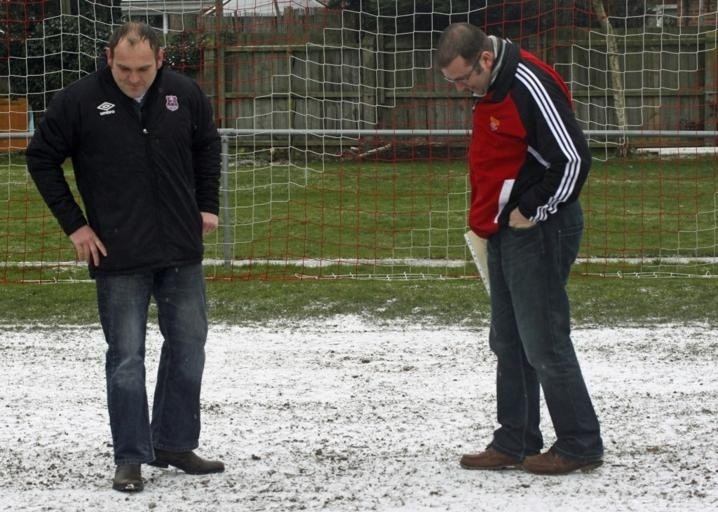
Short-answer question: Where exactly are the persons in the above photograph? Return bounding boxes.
[25,19,226,492]
[436,21,604,474]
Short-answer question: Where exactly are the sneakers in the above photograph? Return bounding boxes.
[522,446,604,475]
[146,447,225,474]
[113,464,144,493]
[460,446,524,471]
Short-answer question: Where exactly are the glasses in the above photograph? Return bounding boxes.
[443,51,483,84]
